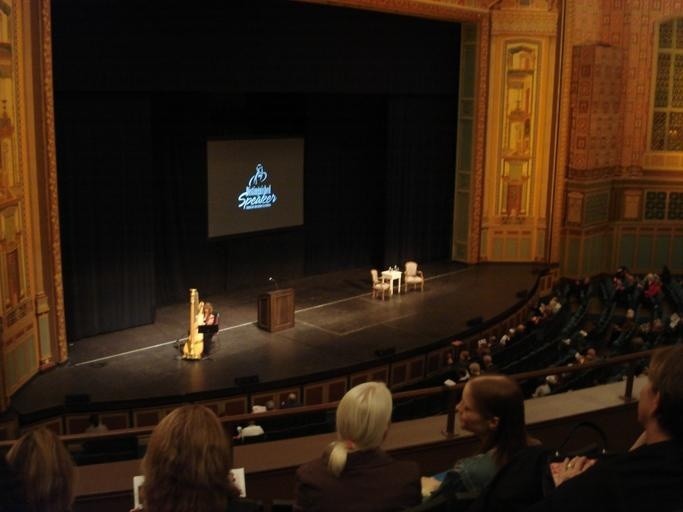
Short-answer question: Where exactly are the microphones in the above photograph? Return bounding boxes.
[269,277,277,283]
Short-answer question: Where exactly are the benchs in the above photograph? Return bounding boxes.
[428,261,682,397]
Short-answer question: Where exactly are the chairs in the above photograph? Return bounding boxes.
[371,269,391,302]
[404,263,425,293]
[208,311,223,349]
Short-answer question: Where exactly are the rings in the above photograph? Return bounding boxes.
[567,463,575,468]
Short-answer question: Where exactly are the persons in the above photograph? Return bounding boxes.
[294,381,423,511]
[201,303,215,326]
[447,263,682,398]
[552,345,682,511]
[418,374,556,511]
[1,426,79,510]
[133,403,265,511]
[241,416,264,442]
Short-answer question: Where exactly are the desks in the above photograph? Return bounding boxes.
[382,269,402,295]
